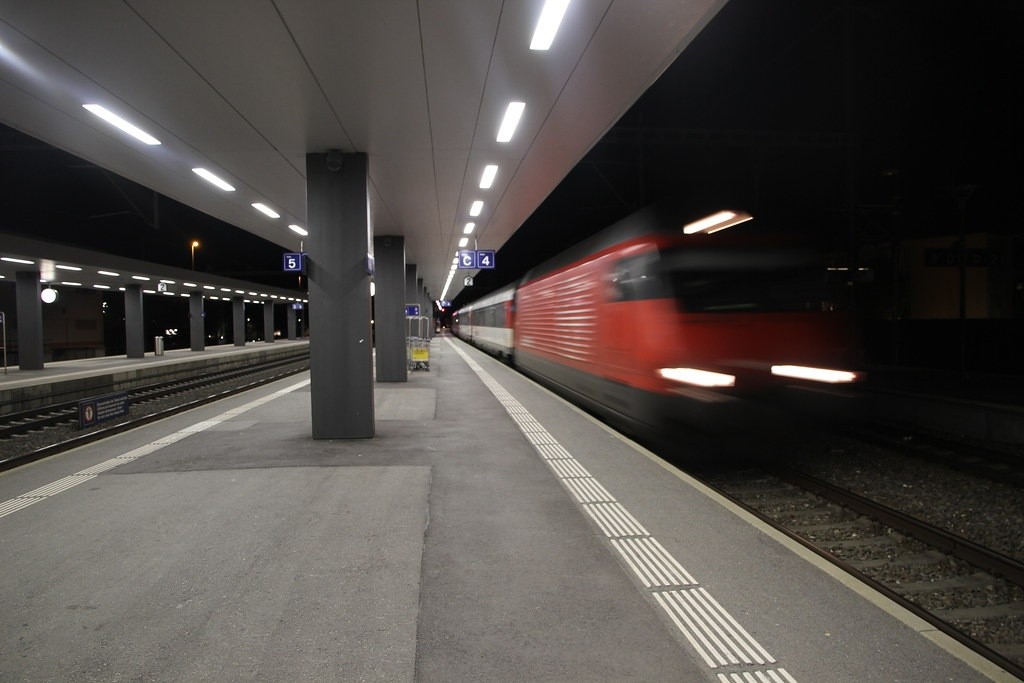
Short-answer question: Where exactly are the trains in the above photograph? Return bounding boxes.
[450,188,868,473]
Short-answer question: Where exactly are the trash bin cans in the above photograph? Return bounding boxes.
[155,336,164,356]
